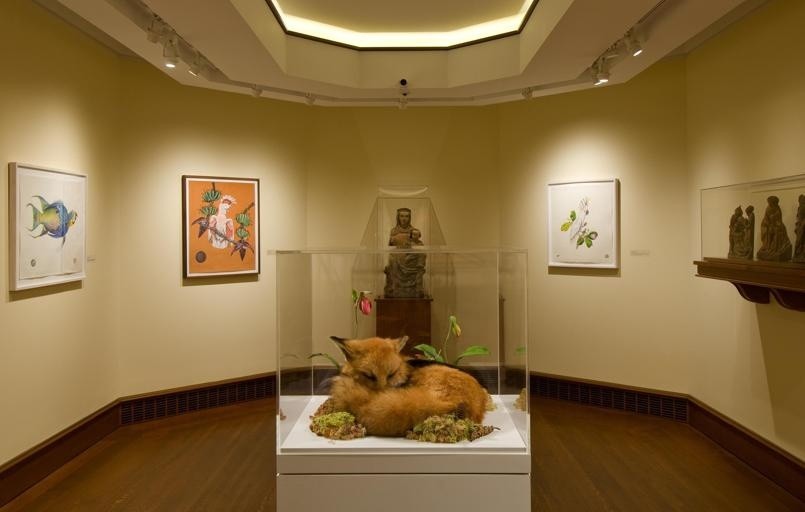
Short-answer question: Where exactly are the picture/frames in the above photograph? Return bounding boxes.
[183,174,260,278]
[8,162,88,293]
[548,180,620,272]
